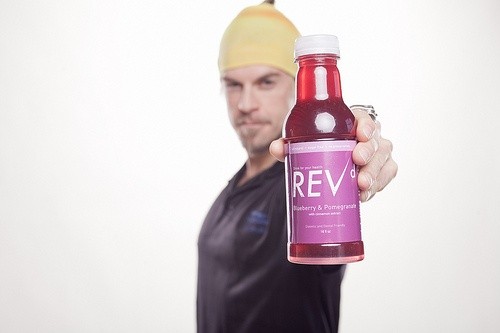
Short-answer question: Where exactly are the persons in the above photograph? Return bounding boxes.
[197,1,398,333]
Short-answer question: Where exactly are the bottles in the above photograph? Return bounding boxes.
[282,32,365,266]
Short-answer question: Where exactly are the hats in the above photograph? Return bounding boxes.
[217,0,300,79]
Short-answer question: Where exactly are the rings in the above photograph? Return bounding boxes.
[349,104,378,119]
